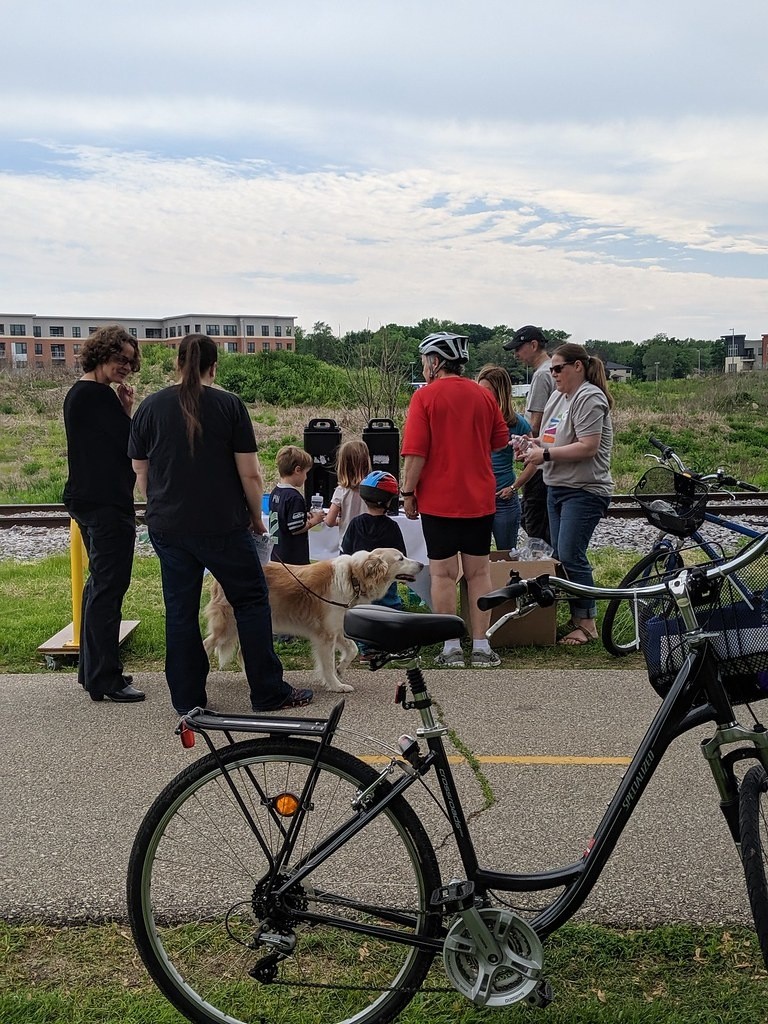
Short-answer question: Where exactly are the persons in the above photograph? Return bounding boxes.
[477,364,539,551]
[269,445,325,650]
[339,471,416,664]
[325,441,371,557]
[62,327,145,703]
[128,334,313,715]
[503,325,557,545]
[508,344,616,646]
[400,331,510,667]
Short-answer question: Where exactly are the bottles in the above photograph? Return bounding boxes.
[310,493,323,513]
[511,434,545,469]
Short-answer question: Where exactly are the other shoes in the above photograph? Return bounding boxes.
[359,654,372,664]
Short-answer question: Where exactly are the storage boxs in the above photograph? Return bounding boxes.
[454,548,569,647]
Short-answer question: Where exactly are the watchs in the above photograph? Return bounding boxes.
[400,487,414,496]
[542,447,550,461]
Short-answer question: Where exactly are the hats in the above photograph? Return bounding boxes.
[502,325,548,351]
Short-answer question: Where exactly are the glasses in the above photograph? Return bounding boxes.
[118,355,134,366]
[549,360,584,374]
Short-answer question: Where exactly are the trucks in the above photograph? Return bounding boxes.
[511,385,531,397]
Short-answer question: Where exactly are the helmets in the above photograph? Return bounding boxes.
[418,331,471,365]
[359,470,398,503]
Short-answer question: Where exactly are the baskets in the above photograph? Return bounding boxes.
[627,550,768,706]
[634,467,708,538]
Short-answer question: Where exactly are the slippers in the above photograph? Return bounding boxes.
[557,618,597,646]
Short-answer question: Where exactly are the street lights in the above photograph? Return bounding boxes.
[410,361,415,383]
[729,328,734,371]
[697,350,700,375]
[655,362,661,381]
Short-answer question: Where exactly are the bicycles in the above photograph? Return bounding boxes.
[127,532,767,1024]
[601,436,768,656]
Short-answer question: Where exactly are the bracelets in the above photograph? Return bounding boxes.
[306,521,313,528]
[510,486,519,495]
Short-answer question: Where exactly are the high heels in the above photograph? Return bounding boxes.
[93,683,145,703]
[83,672,134,691]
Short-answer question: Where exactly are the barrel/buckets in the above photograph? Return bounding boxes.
[261,494,270,515]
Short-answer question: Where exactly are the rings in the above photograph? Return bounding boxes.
[407,515,410,518]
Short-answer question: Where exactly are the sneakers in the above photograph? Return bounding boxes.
[173,699,207,717]
[252,688,313,710]
[471,647,501,667]
[432,647,465,668]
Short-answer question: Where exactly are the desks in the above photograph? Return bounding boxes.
[306,511,463,612]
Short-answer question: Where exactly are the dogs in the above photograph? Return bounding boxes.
[202,547,425,694]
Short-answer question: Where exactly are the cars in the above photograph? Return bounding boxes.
[409,382,428,392]
